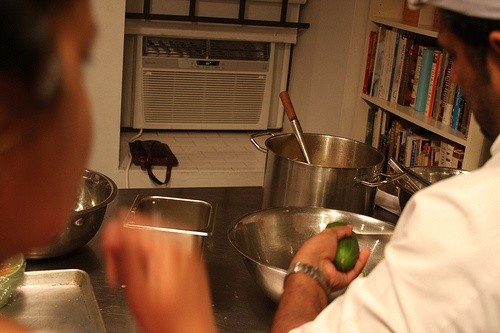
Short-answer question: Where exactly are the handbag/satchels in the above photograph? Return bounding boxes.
[130,139,179,185]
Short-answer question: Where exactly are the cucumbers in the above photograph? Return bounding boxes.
[324,222,359,271]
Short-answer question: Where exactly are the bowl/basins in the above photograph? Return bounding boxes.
[394,166,471,210]
[23,168,117,258]
[123,194,218,257]
[227,205,395,304]
[0,253,26,309]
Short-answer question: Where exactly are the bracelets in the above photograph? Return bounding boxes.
[283,262,331,302]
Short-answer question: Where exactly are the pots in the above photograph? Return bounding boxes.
[250,132,401,217]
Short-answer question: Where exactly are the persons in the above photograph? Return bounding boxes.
[271,0,500,333]
[0,0,219,333]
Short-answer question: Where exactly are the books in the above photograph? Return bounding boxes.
[362,25,473,181]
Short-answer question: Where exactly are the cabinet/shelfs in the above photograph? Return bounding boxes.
[351,0,493,212]
